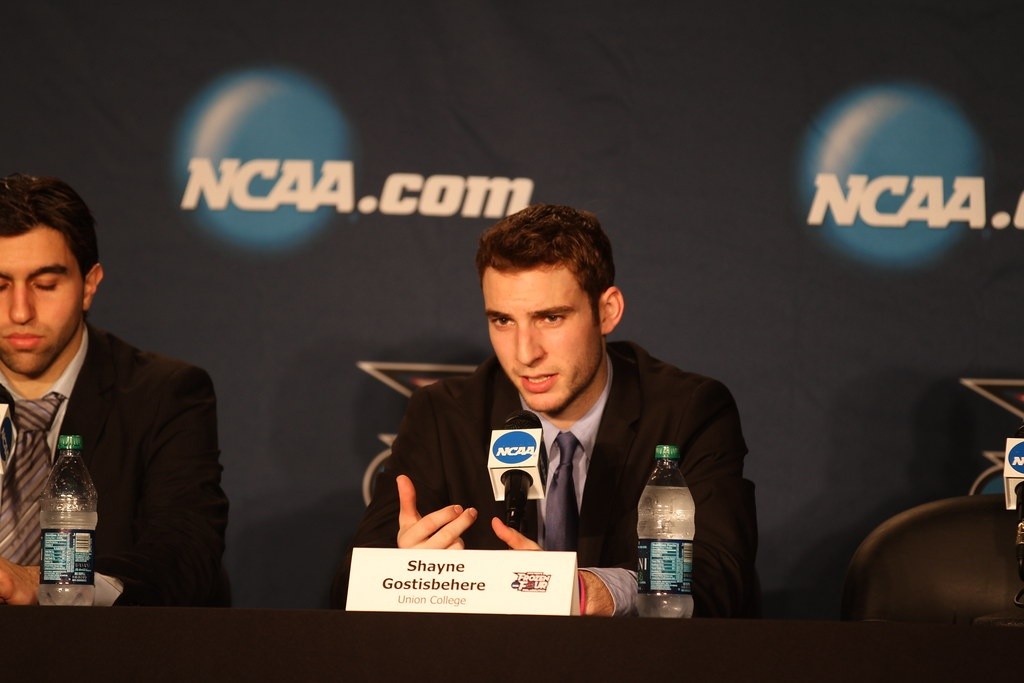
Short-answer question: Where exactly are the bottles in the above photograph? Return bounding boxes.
[37,435,98,607]
[636,444,695,619]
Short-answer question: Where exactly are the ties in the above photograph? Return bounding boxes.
[543,430,580,551]
[0,390,66,567]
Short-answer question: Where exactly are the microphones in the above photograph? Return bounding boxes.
[486,410,550,535]
[0,383,19,475]
[1003,423,1024,558]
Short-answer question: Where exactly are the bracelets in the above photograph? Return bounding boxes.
[578,573,587,616]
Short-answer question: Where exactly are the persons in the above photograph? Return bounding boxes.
[0,168,231,607]
[336,205,761,620]
